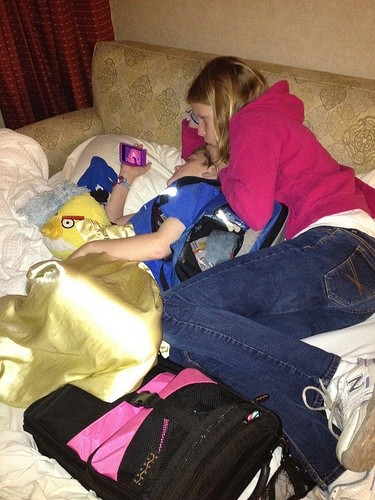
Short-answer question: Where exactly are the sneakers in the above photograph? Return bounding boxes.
[301,357,375,473]
[314,465,375,500]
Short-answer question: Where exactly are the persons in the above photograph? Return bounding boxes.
[65,56,375,500]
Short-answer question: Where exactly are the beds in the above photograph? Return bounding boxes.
[0,40,373,500]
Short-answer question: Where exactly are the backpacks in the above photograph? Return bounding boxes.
[151,175,290,292]
[24,353,283,500]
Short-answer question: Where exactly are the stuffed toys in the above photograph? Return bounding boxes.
[39,192,111,260]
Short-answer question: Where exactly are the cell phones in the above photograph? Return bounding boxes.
[119,142,146,167]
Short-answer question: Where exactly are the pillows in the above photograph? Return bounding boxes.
[60,134,188,218]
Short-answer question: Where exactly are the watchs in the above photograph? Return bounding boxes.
[117,176,131,190]
